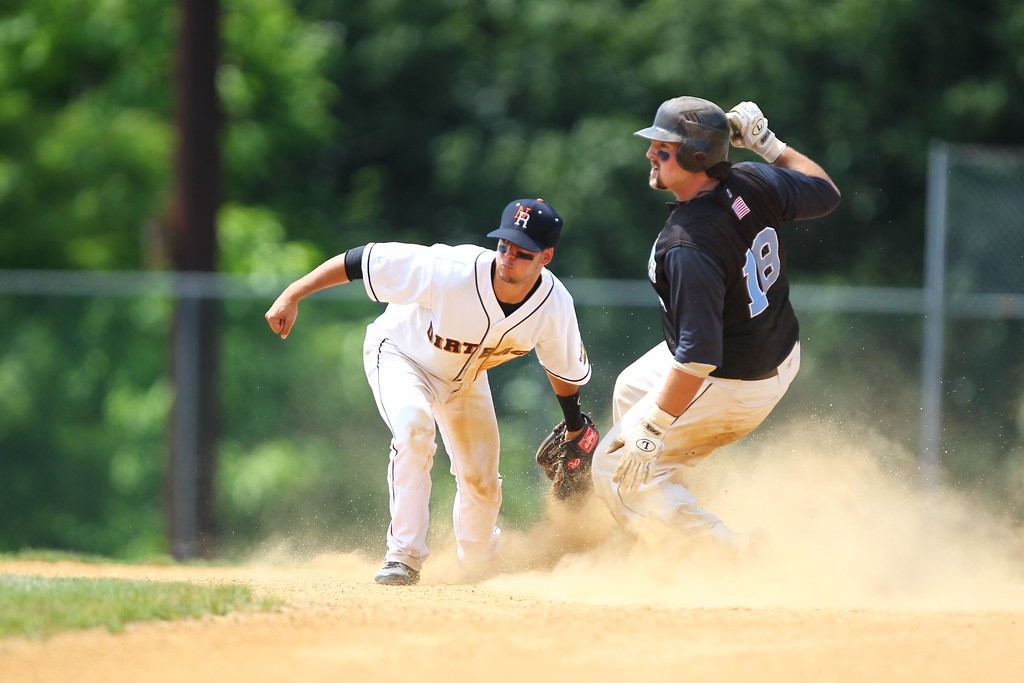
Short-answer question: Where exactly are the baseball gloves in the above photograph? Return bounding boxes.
[534,409,599,501]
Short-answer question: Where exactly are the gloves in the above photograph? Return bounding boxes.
[606,416,666,495]
[726,101,777,155]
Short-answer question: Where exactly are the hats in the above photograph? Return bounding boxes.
[486,198,564,252]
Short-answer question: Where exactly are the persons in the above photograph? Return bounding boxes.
[591,96,842,574]
[263,197,599,586]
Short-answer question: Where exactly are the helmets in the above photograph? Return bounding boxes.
[632,95,732,173]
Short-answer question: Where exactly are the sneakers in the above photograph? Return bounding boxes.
[374,560,421,587]
